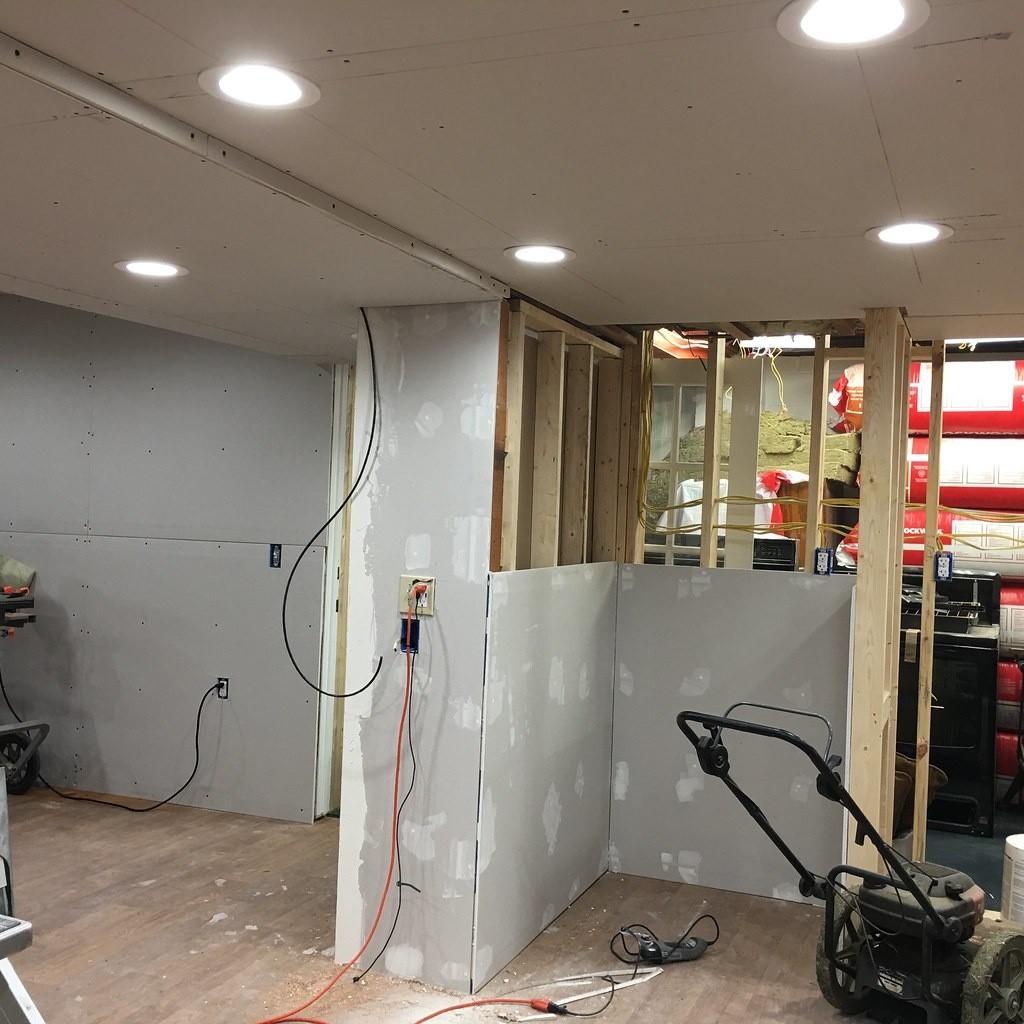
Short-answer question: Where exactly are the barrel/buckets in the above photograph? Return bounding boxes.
[1001,833,1024,932]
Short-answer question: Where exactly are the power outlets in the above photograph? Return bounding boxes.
[219,680,228,697]
[398,575,436,616]
[934,552,953,582]
[814,548,833,576]
